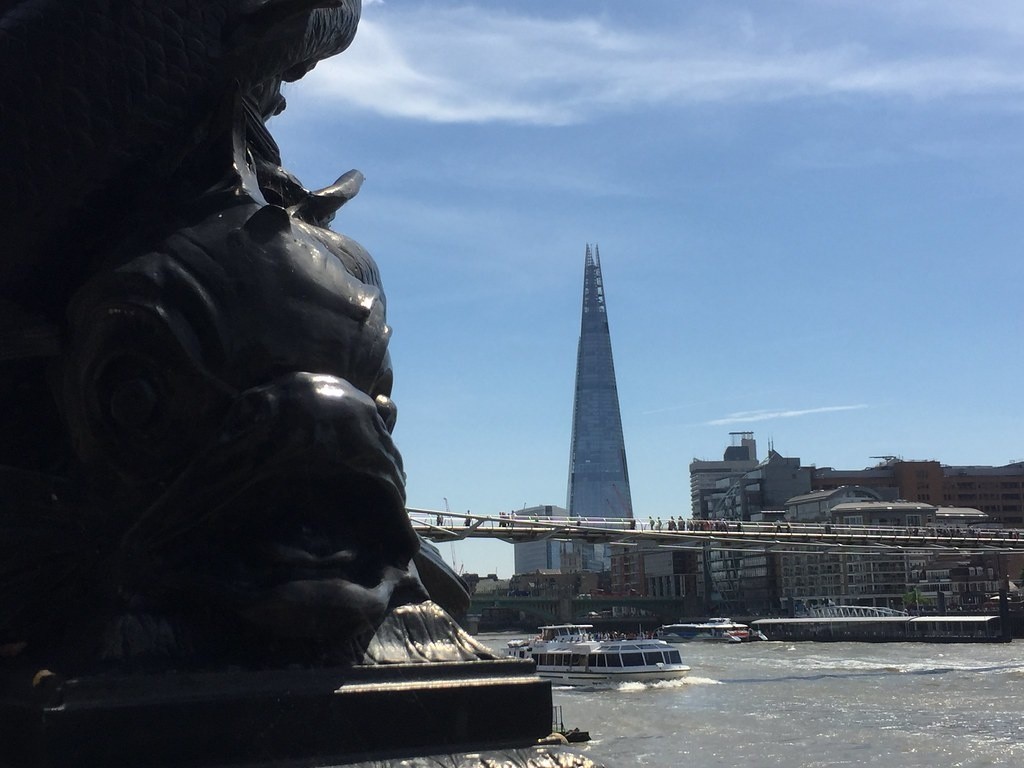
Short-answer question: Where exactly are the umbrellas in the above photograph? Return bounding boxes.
[990,596,1011,601]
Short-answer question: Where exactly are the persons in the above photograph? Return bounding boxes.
[736,519,742,532]
[630,517,635,530]
[464,510,472,526]
[588,630,654,641]
[995,527,999,538]
[668,515,729,532]
[553,636,581,642]
[657,517,662,529]
[499,510,516,527]
[913,524,981,537]
[577,513,581,526]
[437,515,443,526]
[786,522,791,533]
[649,516,655,530]
[776,519,782,533]
[825,522,832,534]
[534,513,538,528]
[1009,528,1020,539]
[582,633,586,641]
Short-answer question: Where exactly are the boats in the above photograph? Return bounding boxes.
[652,617,750,642]
[501,622,692,687]
